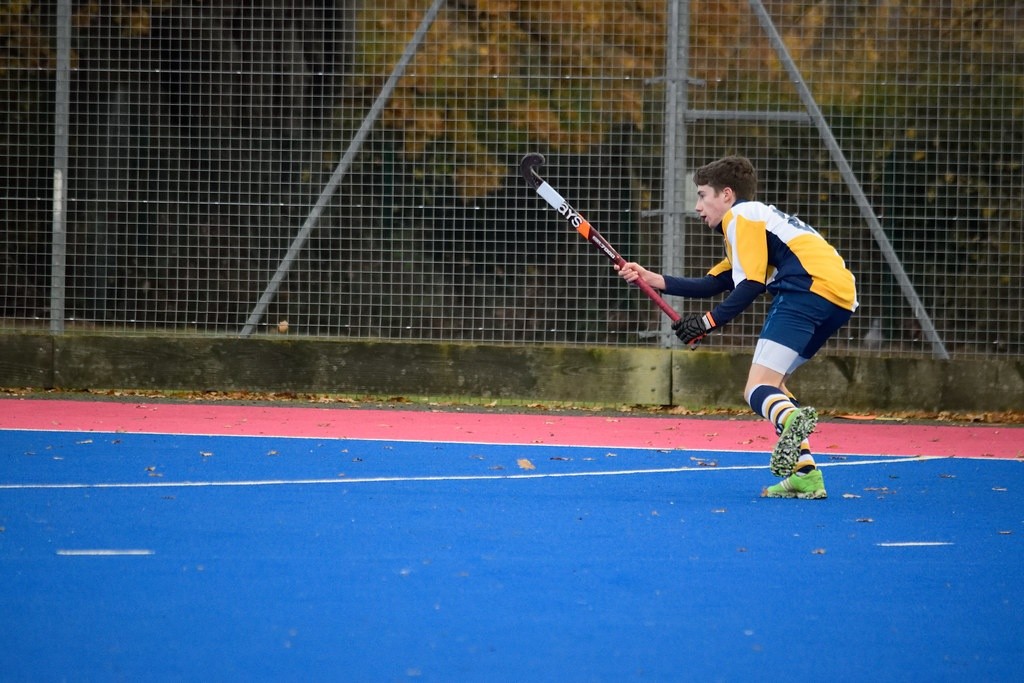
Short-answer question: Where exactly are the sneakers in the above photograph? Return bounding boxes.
[761,469,827,500]
[770,406,818,478]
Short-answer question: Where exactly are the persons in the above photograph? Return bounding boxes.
[613,155,860,499]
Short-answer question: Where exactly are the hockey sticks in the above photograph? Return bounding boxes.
[520,151,702,352]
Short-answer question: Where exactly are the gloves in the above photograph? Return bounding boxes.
[670,311,717,345]
